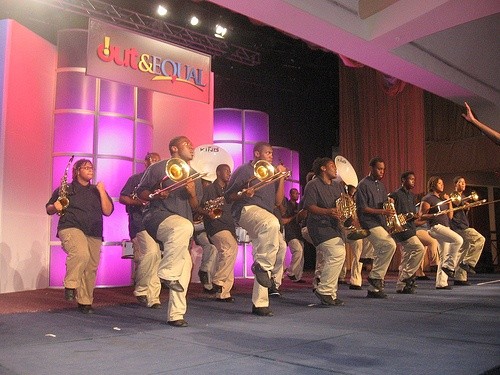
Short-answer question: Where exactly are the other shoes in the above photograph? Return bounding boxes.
[367,276,385,291]
[159,278,184,292]
[150,303,161,308]
[79,303,95,314]
[459,260,476,275]
[397,287,415,294]
[288,274,296,280]
[169,319,189,327]
[269,277,280,295]
[197,270,236,302]
[436,284,451,290]
[137,294,148,305]
[337,281,346,285]
[250,262,272,288]
[298,279,306,283]
[368,290,387,298]
[454,280,472,286]
[321,299,344,306]
[64,287,74,301]
[442,267,455,278]
[349,284,363,290]
[402,277,416,286]
[415,275,430,279]
[313,290,333,304]
[252,305,273,317]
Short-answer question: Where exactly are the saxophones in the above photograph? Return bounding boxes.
[193,195,225,225]
[58,155,75,209]
[129,163,154,200]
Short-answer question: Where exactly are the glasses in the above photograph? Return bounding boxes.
[80,167,95,171]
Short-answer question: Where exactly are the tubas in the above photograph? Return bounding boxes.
[336,190,370,240]
[383,195,408,237]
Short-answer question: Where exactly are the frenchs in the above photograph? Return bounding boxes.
[190,144,234,182]
[334,155,359,188]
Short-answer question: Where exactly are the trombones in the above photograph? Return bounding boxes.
[229,160,290,199]
[419,190,500,223]
[149,156,207,200]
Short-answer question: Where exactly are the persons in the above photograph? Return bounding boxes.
[356,156,395,298]
[136,136,203,328]
[225,141,287,317]
[463,101,500,147]
[119,152,162,309]
[302,156,349,306]
[46,159,114,315]
[117,137,486,327]
